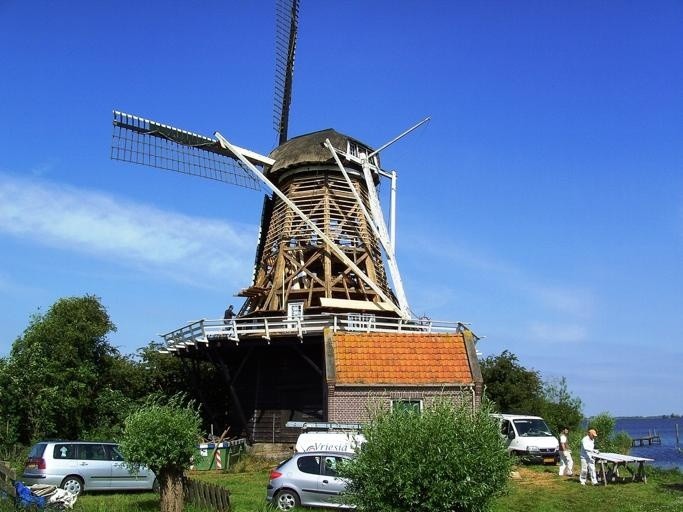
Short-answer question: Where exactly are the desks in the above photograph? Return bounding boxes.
[593,452,654,486]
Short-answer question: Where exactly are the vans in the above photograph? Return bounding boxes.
[486,411,560,466]
[19,439,161,497]
[283,417,366,452]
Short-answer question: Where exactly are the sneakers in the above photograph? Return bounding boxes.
[581,482,601,487]
[558,473,575,477]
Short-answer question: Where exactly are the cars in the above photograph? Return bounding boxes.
[265,450,371,511]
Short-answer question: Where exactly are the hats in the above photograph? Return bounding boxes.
[558,424,568,430]
[589,429,597,437]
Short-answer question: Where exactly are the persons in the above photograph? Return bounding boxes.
[577,427,600,486]
[558,427,574,478]
[223,305,238,331]
